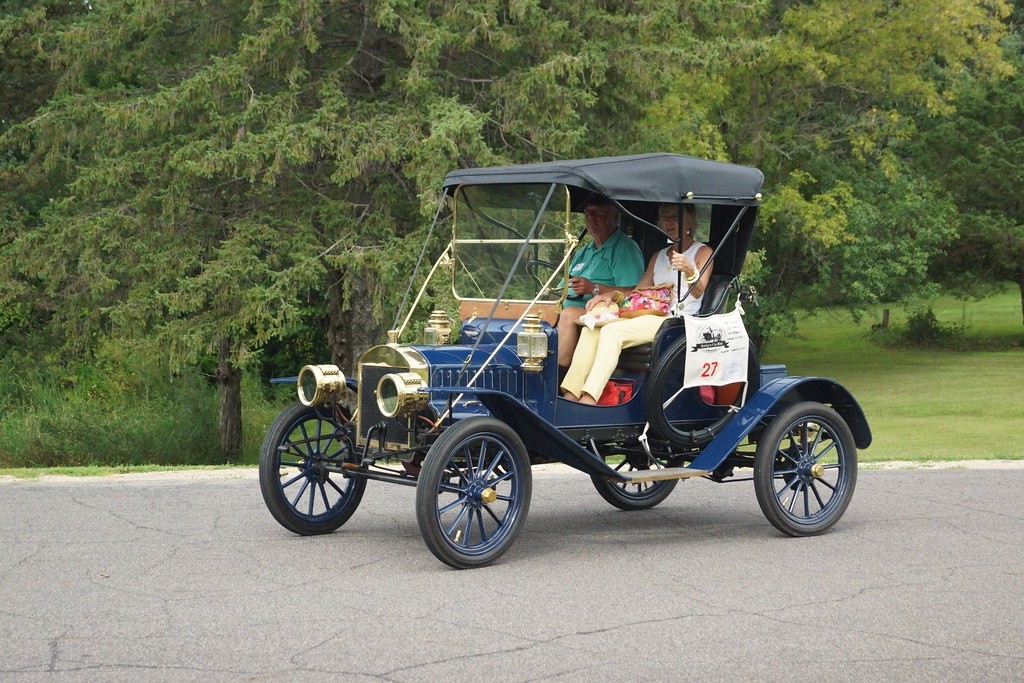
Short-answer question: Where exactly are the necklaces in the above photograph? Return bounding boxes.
[667,238,692,270]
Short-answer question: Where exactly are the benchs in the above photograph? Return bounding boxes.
[608,240,727,371]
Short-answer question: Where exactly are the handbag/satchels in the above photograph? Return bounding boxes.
[619,282,675,318]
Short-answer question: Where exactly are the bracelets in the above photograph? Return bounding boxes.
[684,268,700,284]
[613,290,625,306]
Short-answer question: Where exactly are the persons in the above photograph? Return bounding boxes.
[560,202,713,406]
[556,191,645,396]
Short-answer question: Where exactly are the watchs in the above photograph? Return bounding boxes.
[593,284,600,297]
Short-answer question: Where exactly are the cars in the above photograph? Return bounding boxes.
[258,152,873,569]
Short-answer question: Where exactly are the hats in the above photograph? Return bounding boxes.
[583,192,610,206]
